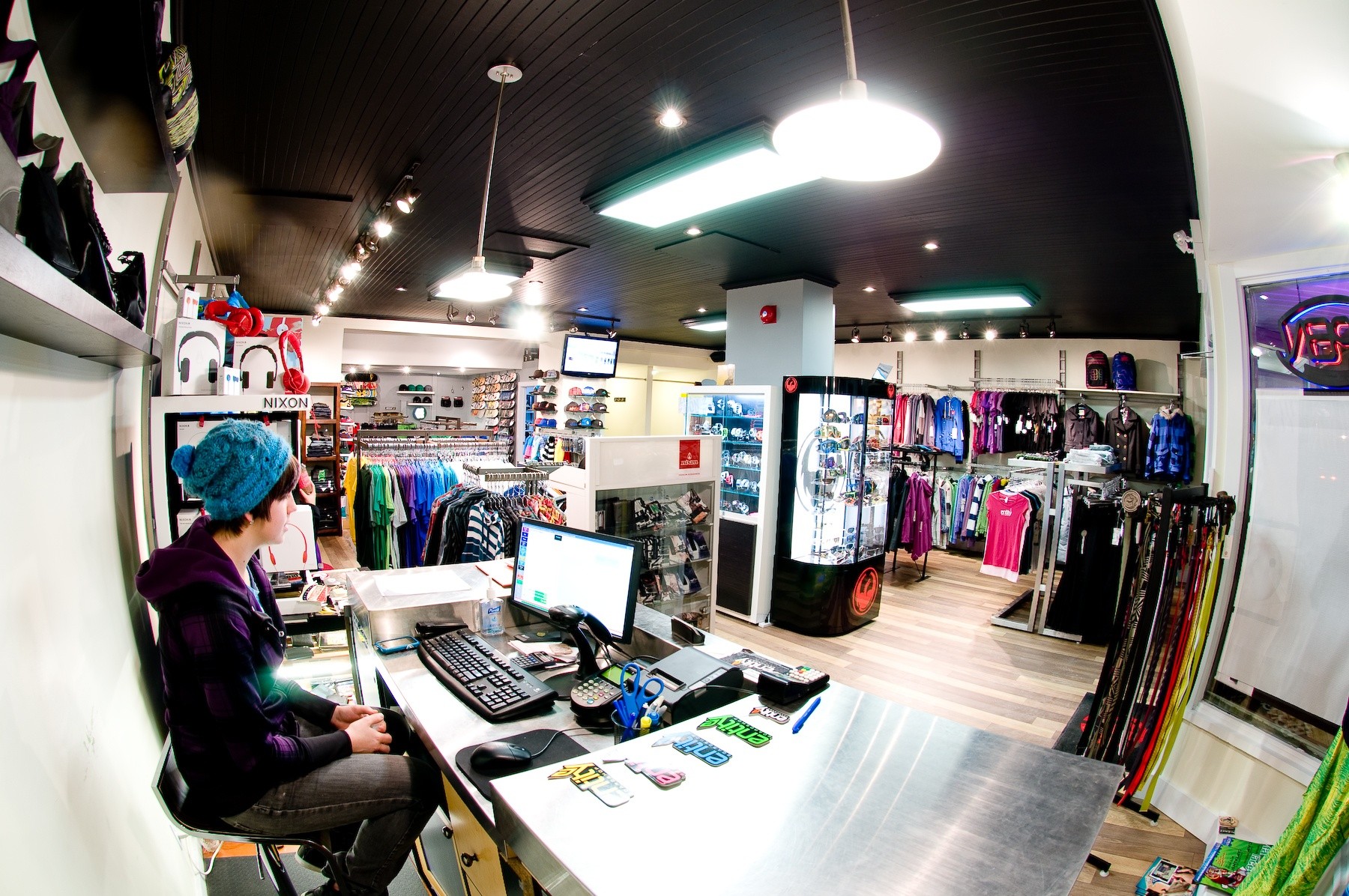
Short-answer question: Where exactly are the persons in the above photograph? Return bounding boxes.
[134,419,443,896]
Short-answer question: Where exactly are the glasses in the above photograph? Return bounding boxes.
[812,409,890,564]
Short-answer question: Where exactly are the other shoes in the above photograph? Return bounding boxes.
[296,843,328,872]
[301,885,336,896]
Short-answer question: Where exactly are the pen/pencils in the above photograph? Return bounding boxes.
[614,701,633,738]
[656,705,667,717]
[635,702,648,728]
[647,703,657,714]
[650,695,664,712]
[619,700,629,722]
[792,696,821,731]
[621,712,635,743]
[507,565,513,569]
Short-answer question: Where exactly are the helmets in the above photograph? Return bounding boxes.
[399,384,408,391]
[408,384,416,391]
[415,385,424,391]
[423,397,432,403]
[413,396,421,403]
[424,385,432,391]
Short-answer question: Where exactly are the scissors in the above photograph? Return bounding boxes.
[621,663,663,728]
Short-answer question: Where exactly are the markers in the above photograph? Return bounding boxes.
[640,716,651,736]
[648,710,660,724]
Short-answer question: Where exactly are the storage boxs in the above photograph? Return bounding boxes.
[162,317,286,395]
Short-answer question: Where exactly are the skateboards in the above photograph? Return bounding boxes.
[344,372,378,406]
[470,372,517,462]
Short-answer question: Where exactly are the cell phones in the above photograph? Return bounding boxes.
[376,636,419,654]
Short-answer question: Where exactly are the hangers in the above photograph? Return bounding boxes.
[892,461,1046,511]
[1106,395,1142,421]
[351,428,596,531]
[1066,395,1098,417]
[1151,399,1192,425]
[897,383,933,406]
[936,385,968,407]
[973,386,1057,401]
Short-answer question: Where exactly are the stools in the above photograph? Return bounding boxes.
[151,729,343,896]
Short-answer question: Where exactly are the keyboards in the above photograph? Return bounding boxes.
[417,627,558,722]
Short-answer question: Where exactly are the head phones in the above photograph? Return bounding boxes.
[278,329,311,394]
[239,343,279,391]
[204,299,268,338]
[268,524,308,565]
[177,331,221,383]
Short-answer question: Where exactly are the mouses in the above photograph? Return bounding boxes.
[470,741,532,776]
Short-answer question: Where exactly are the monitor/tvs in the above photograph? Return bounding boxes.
[510,514,644,699]
[562,333,620,378]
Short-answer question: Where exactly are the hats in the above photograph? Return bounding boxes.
[592,403,609,412]
[171,418,293,519]
[566,402,582,412]
[582,386,597,396]
[569,387,583,395]
[577,418,593,428]
[565,419,580,429]
[529,385,557,428]
[528,369,544,379]
[596,388,610,397]
[590,419,604,429]
[579,403,593,412]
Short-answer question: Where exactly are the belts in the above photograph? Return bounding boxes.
[1075,496,1236,811]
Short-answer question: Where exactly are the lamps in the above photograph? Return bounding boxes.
[314,159,427,325]
[1046,318,1056,339]
[772,0,944,183]
[959,321,971,339]
[438,58,524,303]
[851,326,860,343]
[882,325,892,342]
[1019,318,1029,338]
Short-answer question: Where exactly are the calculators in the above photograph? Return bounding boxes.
[511,651,555,669]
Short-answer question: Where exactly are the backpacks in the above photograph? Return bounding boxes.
[1112,351,1138,391]
[1085,350,1111,389]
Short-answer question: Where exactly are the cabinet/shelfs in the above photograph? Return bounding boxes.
[298,382,342,536]
[340,372,517,443]
[529,375,896,639]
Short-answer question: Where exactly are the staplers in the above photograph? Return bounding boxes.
[414,621,468,641]
